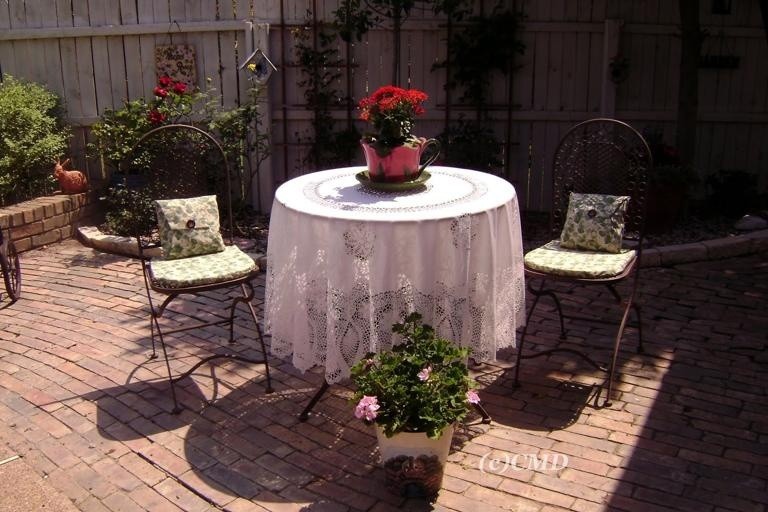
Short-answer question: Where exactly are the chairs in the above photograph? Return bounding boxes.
[513,118,653,407]
[125,124,275,415]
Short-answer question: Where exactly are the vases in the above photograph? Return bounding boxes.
[355,139,442,191]
[368,425,457,503]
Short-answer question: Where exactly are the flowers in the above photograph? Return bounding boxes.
[354,311,480,439]
[359,85,429,145]
